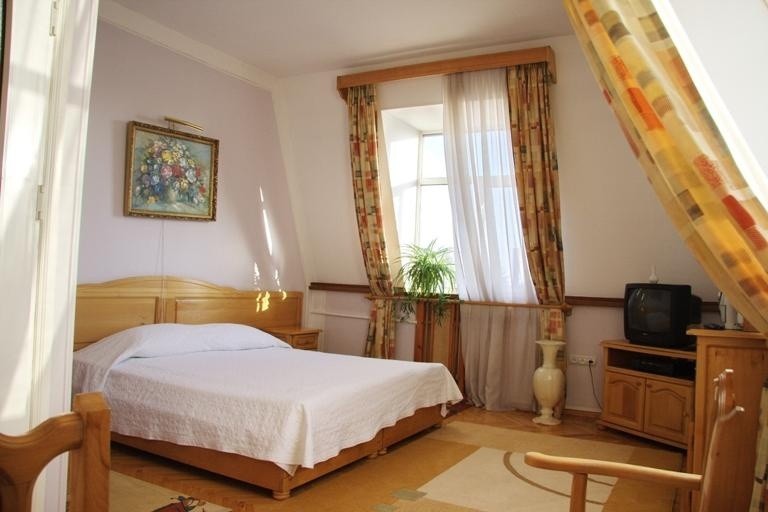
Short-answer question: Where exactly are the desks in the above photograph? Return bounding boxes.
[686,328,768,512]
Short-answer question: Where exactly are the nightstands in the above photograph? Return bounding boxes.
[264,326,323,351]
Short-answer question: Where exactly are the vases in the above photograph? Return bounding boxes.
[530,339,568,426]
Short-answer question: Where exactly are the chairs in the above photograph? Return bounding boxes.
[524,366,746,511]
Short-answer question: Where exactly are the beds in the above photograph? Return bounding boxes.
[73,274,449,501]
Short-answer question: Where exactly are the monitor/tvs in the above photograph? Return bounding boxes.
[624,282,702,349]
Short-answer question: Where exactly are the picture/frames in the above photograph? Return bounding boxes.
[123,119,219,221]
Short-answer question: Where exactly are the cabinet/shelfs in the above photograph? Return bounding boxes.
[598,338,696,456]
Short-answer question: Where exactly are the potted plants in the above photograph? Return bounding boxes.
[387,235,457,329]
